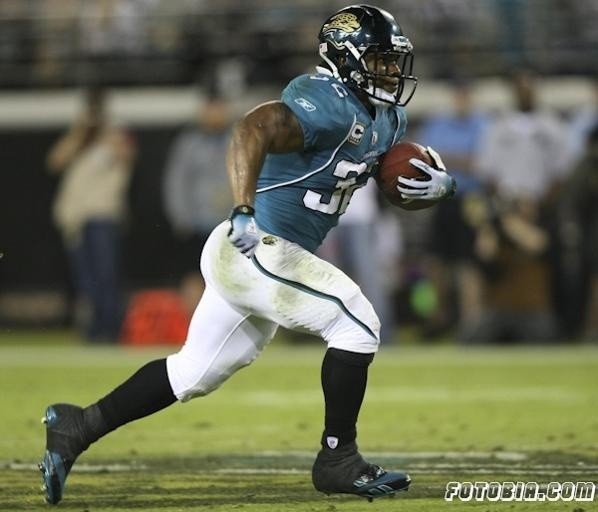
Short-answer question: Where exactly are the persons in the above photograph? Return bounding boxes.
[0,0,598,346]
[35,5,457,504]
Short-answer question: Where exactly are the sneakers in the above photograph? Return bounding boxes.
[38,403,99,505]
[312,441,411,503]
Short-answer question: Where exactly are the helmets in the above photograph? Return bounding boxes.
[317,4,414,88]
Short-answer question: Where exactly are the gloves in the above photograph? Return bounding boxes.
[227,205,261,259]
[396,146,456,202]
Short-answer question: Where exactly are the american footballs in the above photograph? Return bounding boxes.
[381,141,435,211]
